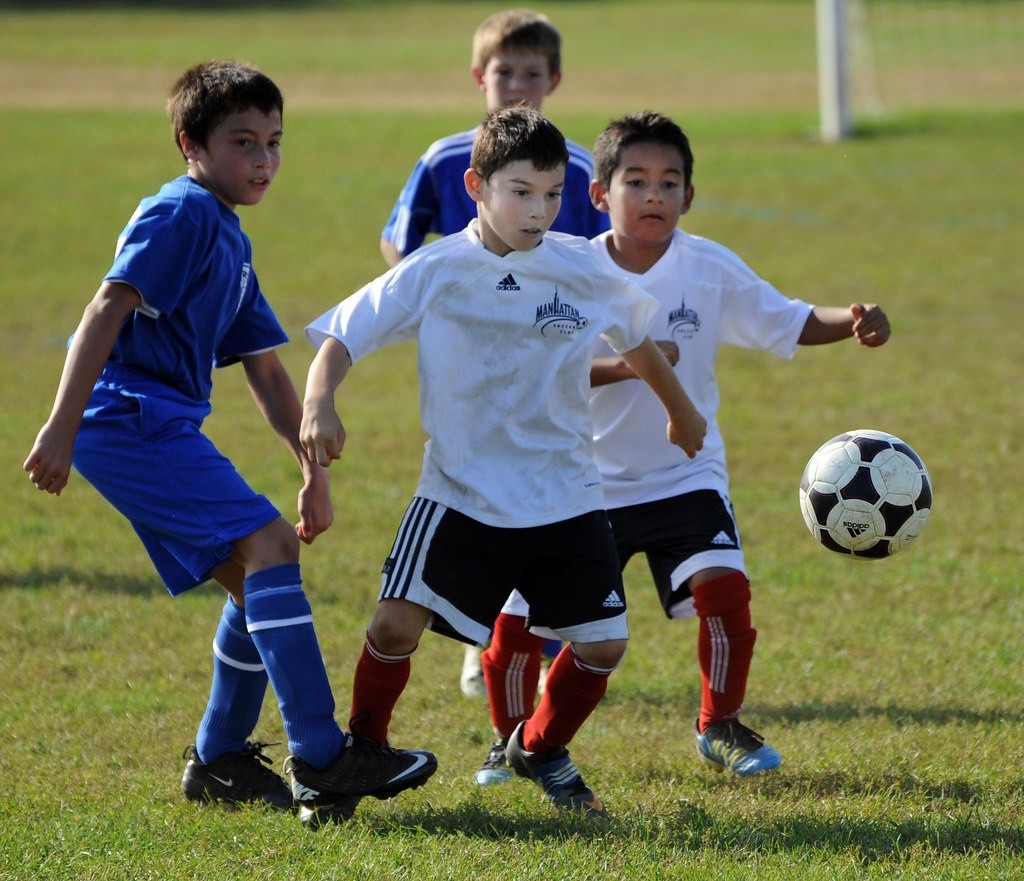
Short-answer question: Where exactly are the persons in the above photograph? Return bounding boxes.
[474,106,892,788]
[378,9,611,700]
[23,61,438,832]
[298,97,707,831]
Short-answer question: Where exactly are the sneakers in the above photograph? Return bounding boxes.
[180,748,295,814]
[473,738,514,785]
[693,716,780,776]
[506,720,607,816]
[297,799,359,832]
[290,735,436,803]
[461,650,488,698]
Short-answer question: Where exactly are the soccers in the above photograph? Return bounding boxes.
[797,427,934,561]
